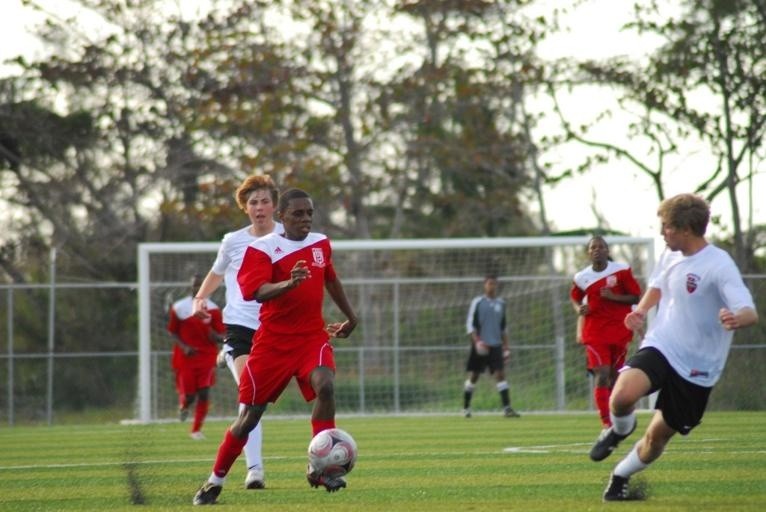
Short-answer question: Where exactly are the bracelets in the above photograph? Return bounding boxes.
[194,295,203,301]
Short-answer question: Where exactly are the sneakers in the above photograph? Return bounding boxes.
[603,470,630,501]
[504,409,520,417]
[306,469,345,493]
[589,418,638,461]
[189,432,204,440]
[216,337,230,369]
[192,481,221,506]
[244,470,264,488]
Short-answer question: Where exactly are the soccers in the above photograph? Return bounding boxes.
[310,427,355,479]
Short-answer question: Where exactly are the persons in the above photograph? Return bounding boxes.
[455,275,520,418]
[569,235,643,428]
[588,193,762,501]
[192,176,287,489]
[166,267,226,441]
[192,188,358,504]
[576,258,638,344]
